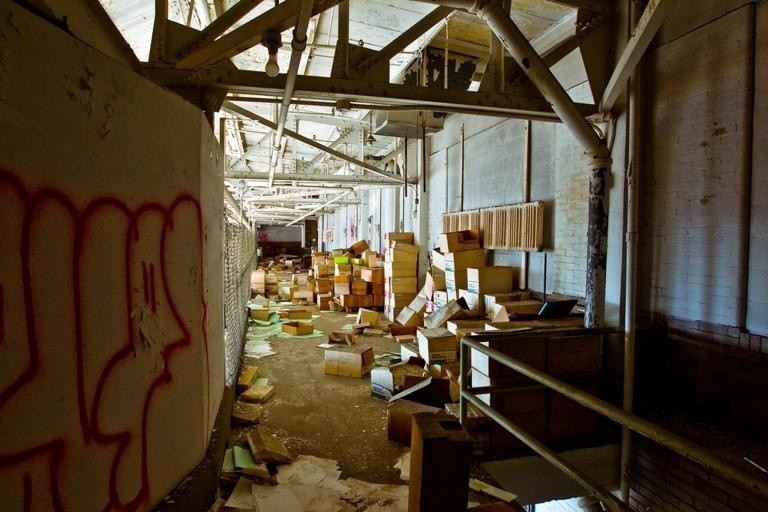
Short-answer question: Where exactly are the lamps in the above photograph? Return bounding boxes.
[262,29,283,78]
